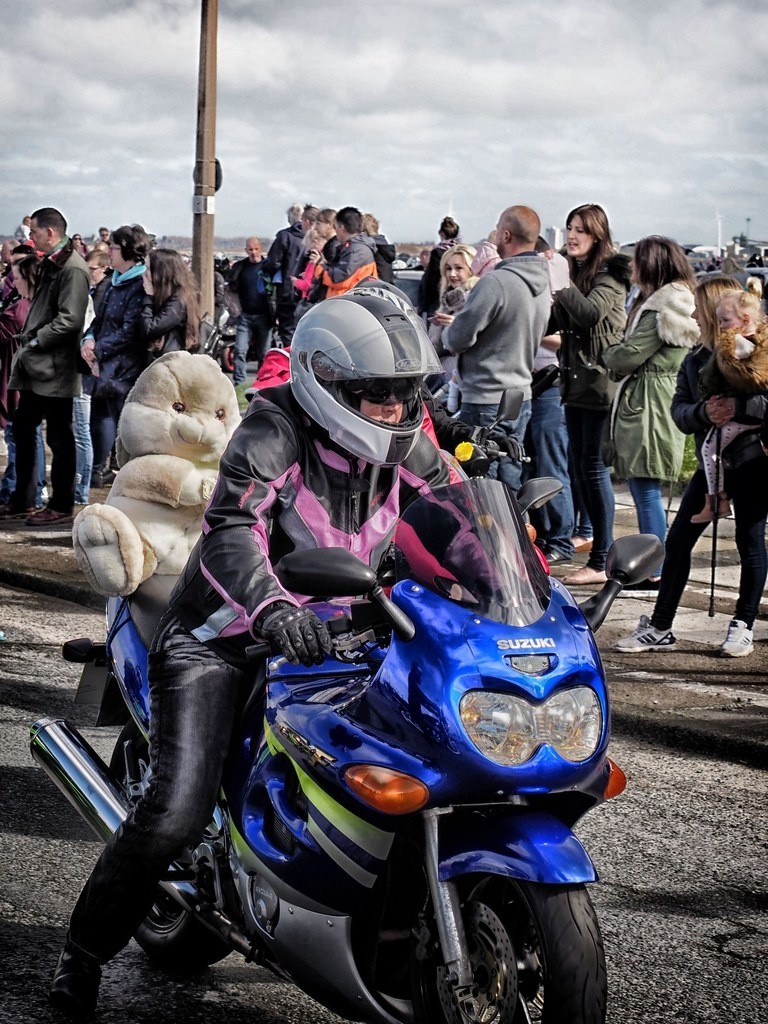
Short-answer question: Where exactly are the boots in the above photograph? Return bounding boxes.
[690,491,732,523]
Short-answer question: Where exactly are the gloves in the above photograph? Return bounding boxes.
[254,601,331,667]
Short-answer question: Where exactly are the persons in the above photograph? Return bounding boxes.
[0,205,768,657]
[47,293,519,1024]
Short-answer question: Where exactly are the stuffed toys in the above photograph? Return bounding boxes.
[72,351,243,599]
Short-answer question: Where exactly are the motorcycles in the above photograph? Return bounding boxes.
[27,474,666,1023]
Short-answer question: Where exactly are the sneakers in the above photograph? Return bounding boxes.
[721,619,754,657]
[611,615,677,652]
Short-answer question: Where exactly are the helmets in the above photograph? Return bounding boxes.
[345,276,428,336]
[290,293,446,467]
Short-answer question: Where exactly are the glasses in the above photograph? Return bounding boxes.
[345,378,424,404]
[75,238,82,240]
[102,234,109,236]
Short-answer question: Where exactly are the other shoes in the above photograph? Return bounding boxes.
[25,507,74,526]
[560,565,608,584]
[51,942,101,1011]
[617,579,661,598]
[574,541,593,554]
[90,472,104,488]
[540,545,573,565]
[102,468,120,487]
[0,501,35,520]
